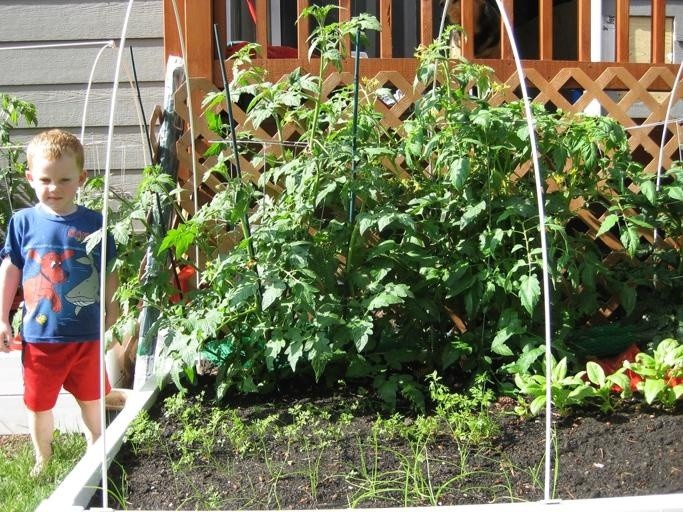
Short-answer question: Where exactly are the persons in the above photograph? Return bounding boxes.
[0,127,121,478]
